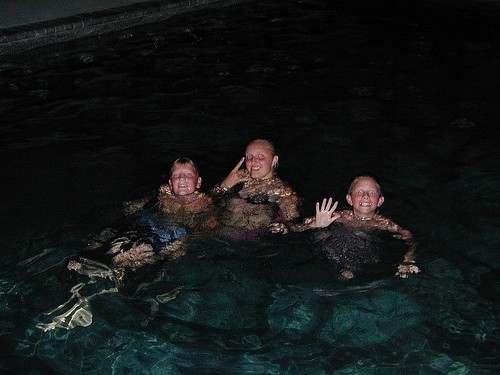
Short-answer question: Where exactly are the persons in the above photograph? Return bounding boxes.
[67,156,216,280]
[209,138,300,241]
[304,175,419,279]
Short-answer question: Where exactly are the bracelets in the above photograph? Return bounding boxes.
[221,181,230,190]
